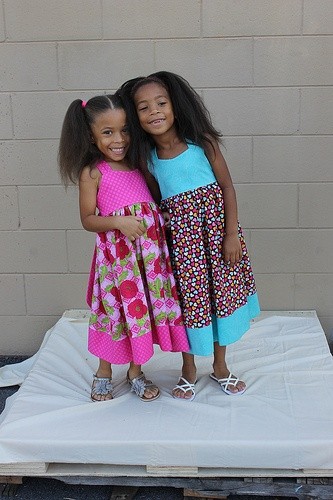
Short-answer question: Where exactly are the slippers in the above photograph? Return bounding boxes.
[209,369,247,396]
[172,372,199,401]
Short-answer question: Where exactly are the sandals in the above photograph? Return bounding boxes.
[127,372,161,402]
[91,372,114,403]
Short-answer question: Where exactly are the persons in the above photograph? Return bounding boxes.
[112,72,261,401]
[58,93,189,404]
